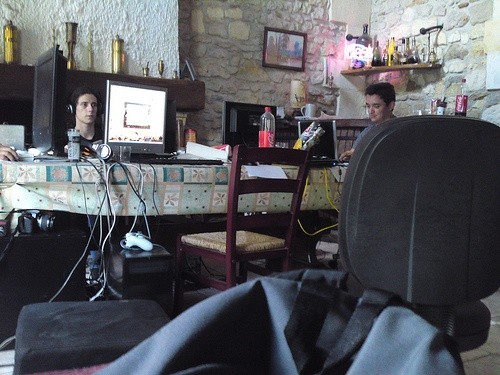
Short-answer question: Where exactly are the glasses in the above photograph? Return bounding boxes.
[363,104,390,109]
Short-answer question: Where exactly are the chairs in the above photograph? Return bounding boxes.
[339,115,500,375]
[173,143,315,316]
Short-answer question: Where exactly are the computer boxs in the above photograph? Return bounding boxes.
[105,244,173,320]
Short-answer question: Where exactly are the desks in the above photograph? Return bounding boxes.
[0,150,348,256]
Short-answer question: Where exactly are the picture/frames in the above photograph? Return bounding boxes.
[262,26,307,72]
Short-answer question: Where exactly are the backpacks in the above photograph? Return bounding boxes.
[87,268,465,375]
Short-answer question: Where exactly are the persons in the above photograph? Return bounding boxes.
[339,82,397,161]
[0,143,19,162]
[53,87,104,156]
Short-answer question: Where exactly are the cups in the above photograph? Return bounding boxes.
[120,146,131,164]
[436,107,444,115]
[300,103,317,117]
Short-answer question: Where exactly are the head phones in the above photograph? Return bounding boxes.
[67,87,102,115]
[78,135,113,160]
[17,209,57,234]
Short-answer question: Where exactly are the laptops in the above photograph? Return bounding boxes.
[298,120,349,163]
[104,80,223,165]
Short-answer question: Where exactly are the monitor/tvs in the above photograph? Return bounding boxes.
[29,44,73,157]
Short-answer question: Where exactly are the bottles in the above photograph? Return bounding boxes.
[257,106,276,165]
[68,129,81,163]
[357,23,420,66]
[455,79,469,117]
